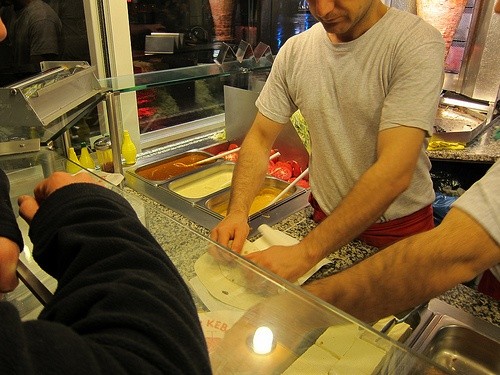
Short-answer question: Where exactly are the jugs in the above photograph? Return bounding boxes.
[95,135,115,173]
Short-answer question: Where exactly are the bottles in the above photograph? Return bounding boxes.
[65,145,81,173]
[122,129,137,165]
[79,142,95,169]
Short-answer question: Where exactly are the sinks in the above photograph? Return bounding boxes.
[370,297,500,375]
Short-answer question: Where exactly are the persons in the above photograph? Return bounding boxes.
[208,0,445,264]
[0,171,25,293]
[222,158,500,374]
[0,0,170,86]
[0,171,215,375]
